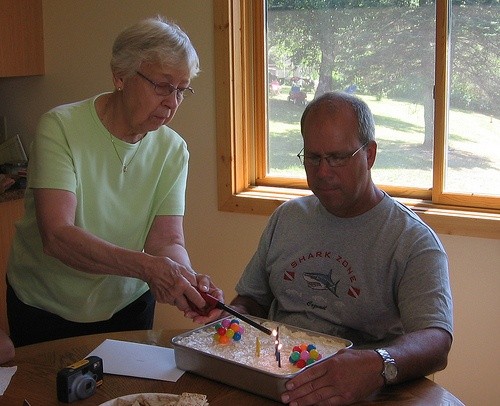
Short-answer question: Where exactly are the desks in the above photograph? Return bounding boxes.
[0,328,466,406]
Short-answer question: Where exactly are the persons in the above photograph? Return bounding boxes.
[219,91,454,406]
[290,83,306,104]
[5,20,225,347]
[345,81,357,94]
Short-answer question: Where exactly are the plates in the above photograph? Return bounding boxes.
[98,392,179,406]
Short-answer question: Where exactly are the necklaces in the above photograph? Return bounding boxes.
[106,97,143,173]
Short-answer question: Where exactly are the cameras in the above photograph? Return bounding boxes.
[55,355,103,404]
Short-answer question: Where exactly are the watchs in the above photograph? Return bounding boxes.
[375,347,398,387]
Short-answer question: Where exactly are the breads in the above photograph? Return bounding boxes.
[116,392,210,406]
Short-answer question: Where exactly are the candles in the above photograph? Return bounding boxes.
[275,327,281,368]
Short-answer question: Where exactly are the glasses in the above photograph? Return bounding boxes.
[297,143,367,168]
[134,68,194,100]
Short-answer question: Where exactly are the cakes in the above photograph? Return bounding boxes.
[176,316,346,375]
